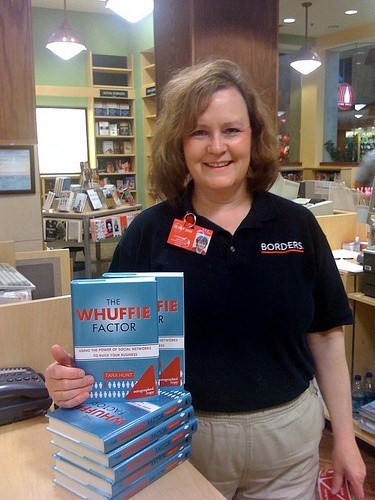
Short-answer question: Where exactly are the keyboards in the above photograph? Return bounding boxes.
[0,263,37,292]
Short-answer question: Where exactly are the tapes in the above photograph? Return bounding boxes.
[318,468,355,500]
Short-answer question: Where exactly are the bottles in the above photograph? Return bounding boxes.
[353,236,360,252]
[351,374,366,421]
[364,371,375,405]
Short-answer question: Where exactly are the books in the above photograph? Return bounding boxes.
[45,272,199,500]
[94,101,133,172]
[42,162,143,243]
[360,400,375,435]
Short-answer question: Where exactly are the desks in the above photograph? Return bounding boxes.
[0,239,227,500]
[41,204,143,278]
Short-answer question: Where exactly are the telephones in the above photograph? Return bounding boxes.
[0,367,53,426]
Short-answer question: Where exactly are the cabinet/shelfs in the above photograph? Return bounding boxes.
[273,162,375,447]
[40,46,167,214]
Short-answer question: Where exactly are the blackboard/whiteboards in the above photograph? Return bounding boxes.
[36,106,89,174]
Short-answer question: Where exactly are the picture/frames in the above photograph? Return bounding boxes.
[0,145,35,195]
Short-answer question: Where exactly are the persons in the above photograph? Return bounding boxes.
[352,147,375,213]
[191,236,208,255]
[44,58,366,500]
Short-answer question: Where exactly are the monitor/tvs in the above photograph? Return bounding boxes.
[265,172,300,202]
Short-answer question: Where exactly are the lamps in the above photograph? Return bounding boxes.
[104,0,154,23]
[350,43,366,118]
[46,0,87,61]
[290,2,322,75]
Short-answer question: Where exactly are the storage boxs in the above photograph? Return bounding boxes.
[362,248,375,298]
